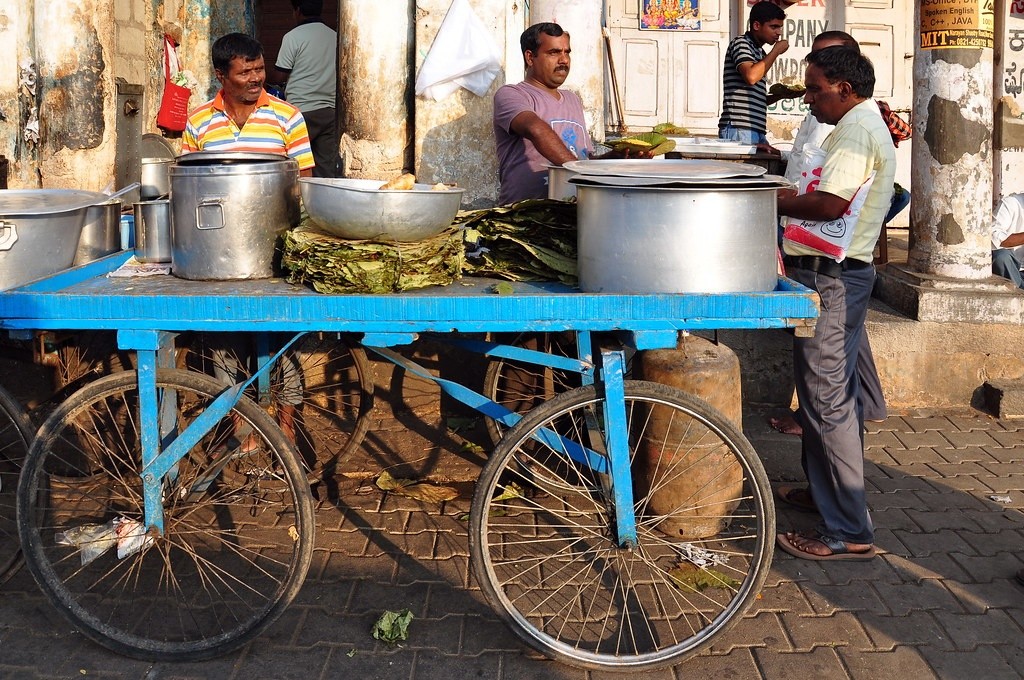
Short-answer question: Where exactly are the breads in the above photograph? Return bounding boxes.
[379,172,450,190]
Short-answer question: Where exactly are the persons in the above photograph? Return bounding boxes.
[718,0,807,145]
[180,32,316,476]
[991,192,1024,290]
[754,31,887,436]
[263,0,336,179]
[776,44,897,562]
[494,22,656,499]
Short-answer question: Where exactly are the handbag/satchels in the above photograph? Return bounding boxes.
[783,141,876,263]
[156,34,191,131]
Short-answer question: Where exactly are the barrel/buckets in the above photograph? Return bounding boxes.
[132,200,171,265]
[72,195,124,268]
[166,150,296,280]
[138,158,172,198]
[541,162,579,202]
[566,175,791,295]
[121,215,134,250]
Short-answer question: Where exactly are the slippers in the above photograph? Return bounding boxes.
[778,486,819,512]
[210,446,259,461]
[274,462,285,475]
[777,528,875,560]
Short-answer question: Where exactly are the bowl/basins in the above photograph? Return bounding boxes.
[296,174,468,240]
[0,188,110,294]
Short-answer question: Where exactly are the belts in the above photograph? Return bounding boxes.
[783,255,868,278]
